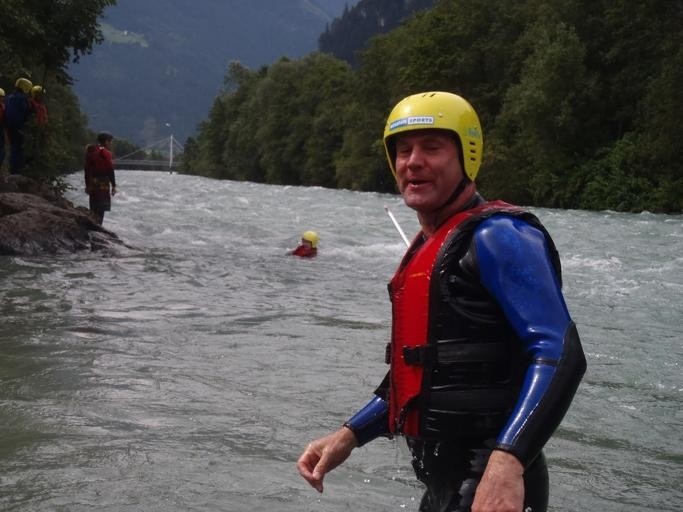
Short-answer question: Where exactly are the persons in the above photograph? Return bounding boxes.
[84,131,116,224]
[4,77,32,175]
[296,89,588,512]
[287,231,319,261]
[31,85,49,126]
[0,87,8,163]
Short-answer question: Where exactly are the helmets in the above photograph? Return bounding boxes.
[15,78,33,94]
[0,88,4,95]
[302,230,320,248]
[31,85,45,95]
[97,131,114,144]
[384,91,484,183]
